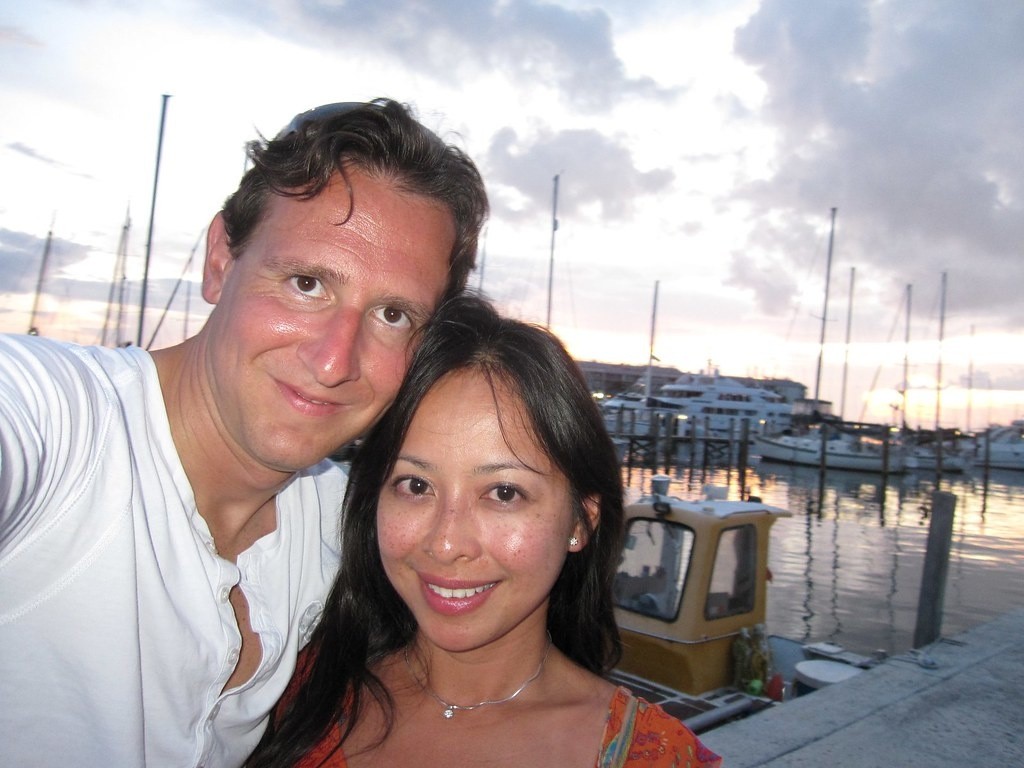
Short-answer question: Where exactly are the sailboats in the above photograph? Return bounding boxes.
[755,206,1024,475]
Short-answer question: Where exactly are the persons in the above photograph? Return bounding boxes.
[239,294,724,768]
[0,99,489,768]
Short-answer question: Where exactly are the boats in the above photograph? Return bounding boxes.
[607,474,886,735]
[600,360,792,444]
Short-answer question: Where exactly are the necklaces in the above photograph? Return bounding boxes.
[403,630,553,721]
